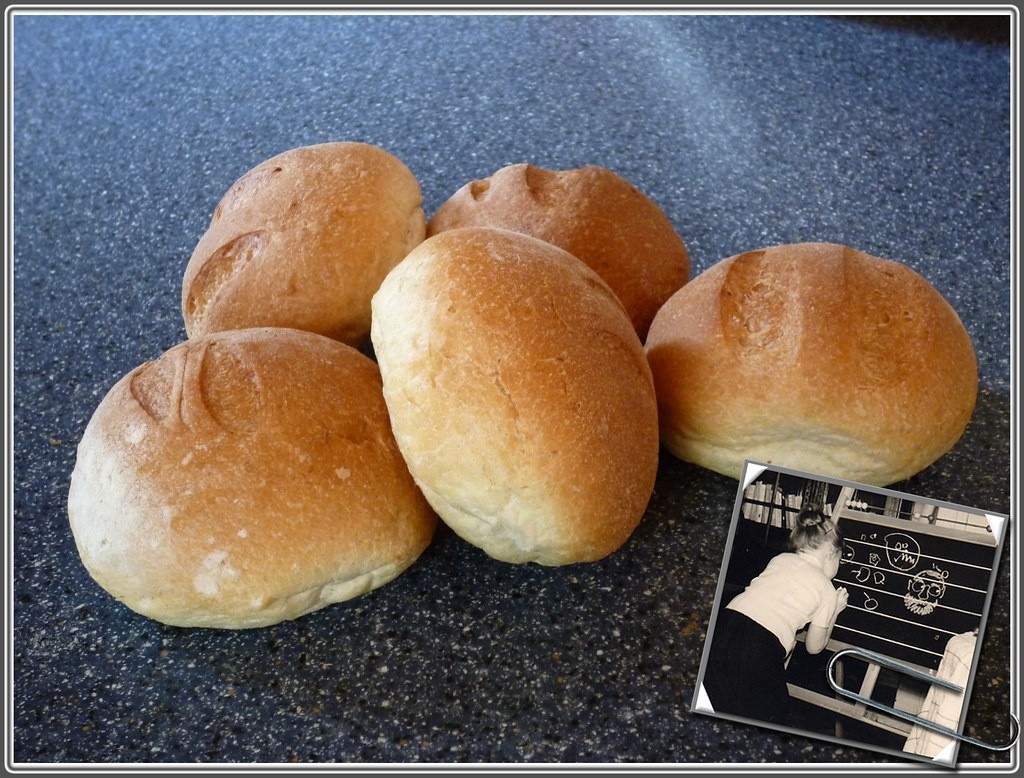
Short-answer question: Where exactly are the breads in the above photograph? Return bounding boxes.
[67,326,435,629]
[642,243,976,487]
[429,164,691,346]
[179,141,427,348]
[370,226,659,565]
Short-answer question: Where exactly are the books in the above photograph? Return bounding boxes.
[743,480,802,530]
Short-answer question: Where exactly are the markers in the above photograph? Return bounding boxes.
[837,586,843,591]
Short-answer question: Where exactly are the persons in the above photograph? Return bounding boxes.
[704,502,849,725]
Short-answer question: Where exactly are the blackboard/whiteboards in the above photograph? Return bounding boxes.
[794,509,1000,690]
